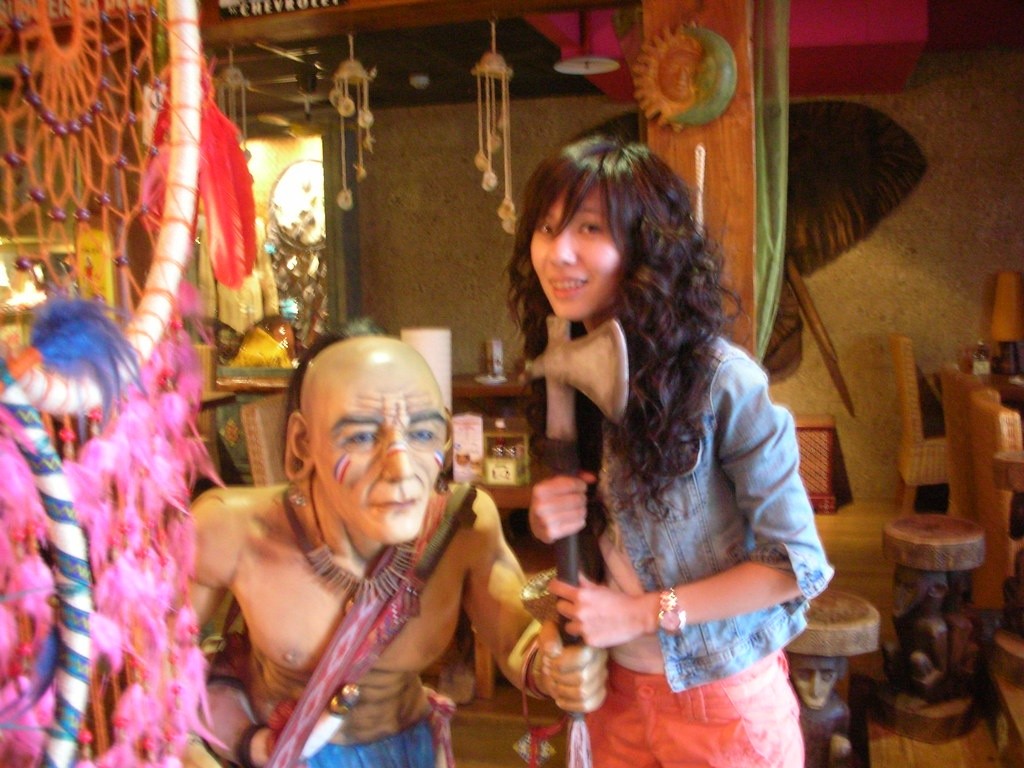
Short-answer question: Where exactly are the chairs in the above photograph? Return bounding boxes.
[966,385,1024,611]
[937,358,988,521]
[884,331,947,519]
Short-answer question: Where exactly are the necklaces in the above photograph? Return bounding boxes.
[278,491,419,604]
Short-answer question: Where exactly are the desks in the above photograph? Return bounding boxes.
[450,369,532,698]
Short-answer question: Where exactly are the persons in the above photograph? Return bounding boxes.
[165,323,608,768]
[503,135,834,768]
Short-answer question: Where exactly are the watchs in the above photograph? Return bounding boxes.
[658,587,683,636]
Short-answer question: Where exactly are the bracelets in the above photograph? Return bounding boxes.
[520,643,569,768]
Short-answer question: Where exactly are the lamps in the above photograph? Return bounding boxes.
[553,10,619,74]
